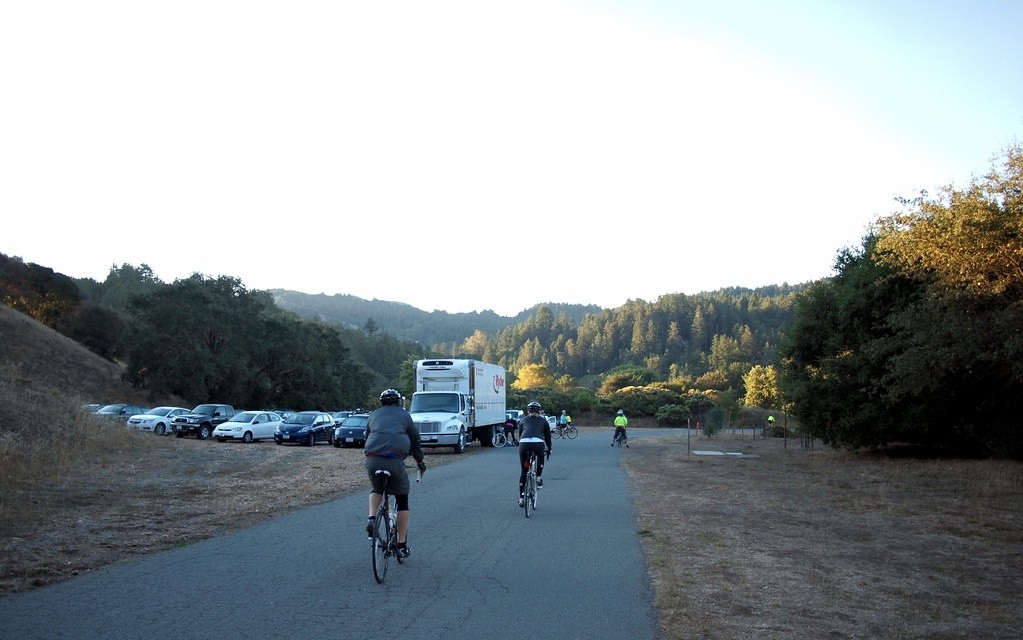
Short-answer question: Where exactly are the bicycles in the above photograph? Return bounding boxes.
[550,425,578,439]
[521,449,550,518]
[492,426,510,448]
[372,466,421,583]
[616,431,626,448]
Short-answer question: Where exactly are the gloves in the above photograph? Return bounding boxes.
[415,462,427,471]
[546,449,552,457]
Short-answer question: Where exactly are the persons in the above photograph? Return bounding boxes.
[505,419,517,443]
[611,409,629,448]
[768,415,775,425]
[519,402,552,505]
[561,410,574,439]
[364,389,426,559]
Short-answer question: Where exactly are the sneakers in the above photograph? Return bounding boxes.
[517,498,524,508]
[536,476,543,487]
[365,519,380,538]
[397,546,412,558]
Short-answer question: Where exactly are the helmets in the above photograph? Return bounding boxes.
[616,412,622,415]
[526,401,541,410]
[617,409,623,412]
[379,389,402,404]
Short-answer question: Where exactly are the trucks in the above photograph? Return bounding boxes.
[409,358,506,454]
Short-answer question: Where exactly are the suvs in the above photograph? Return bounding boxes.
[506,410,524,428]
[170,404,236,439]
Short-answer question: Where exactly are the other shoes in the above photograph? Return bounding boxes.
[626,445,629,448]
[610,444,613,446]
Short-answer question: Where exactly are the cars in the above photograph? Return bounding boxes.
[334,414,370,448]
[212,411,286,443]
[538,413,556,431]
[273,411,336,446]
[81,404,191,436]
